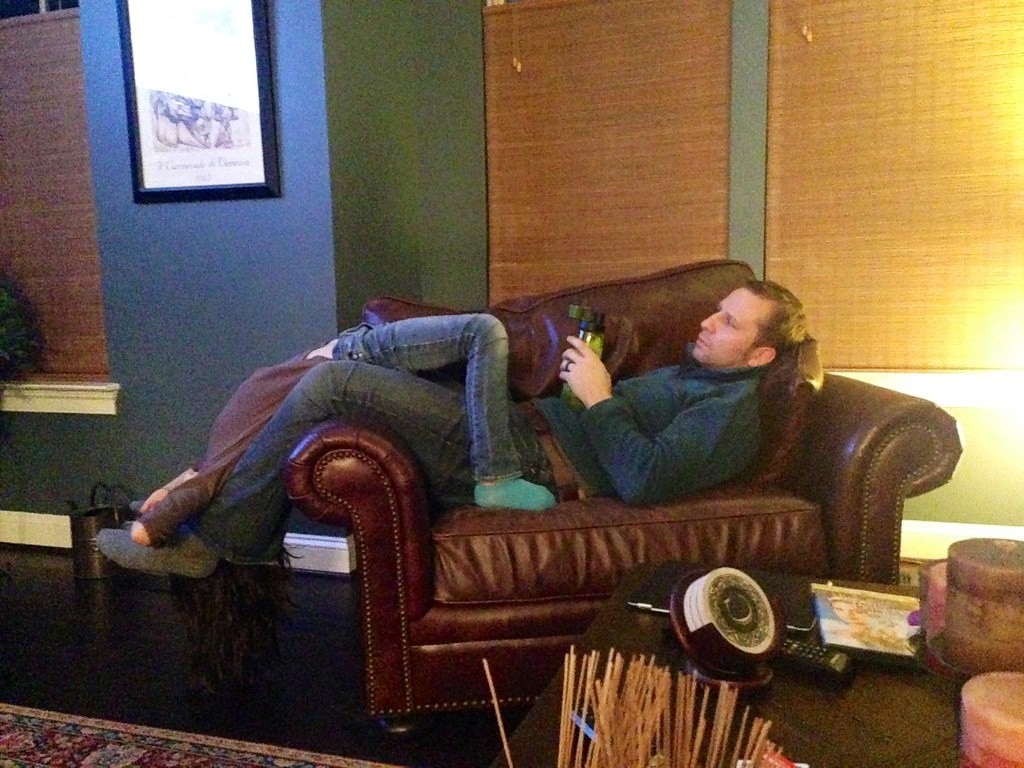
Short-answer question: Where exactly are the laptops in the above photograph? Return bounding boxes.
[625,558,835,631]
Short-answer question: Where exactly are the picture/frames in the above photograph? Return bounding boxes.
[115,1,282,205]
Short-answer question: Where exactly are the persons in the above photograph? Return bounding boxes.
[94,278,814,570]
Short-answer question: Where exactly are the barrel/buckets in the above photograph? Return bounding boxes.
[70,505,119,580]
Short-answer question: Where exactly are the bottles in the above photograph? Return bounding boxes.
[560,308,606,407]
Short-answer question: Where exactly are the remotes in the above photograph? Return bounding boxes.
[773,636,858,686]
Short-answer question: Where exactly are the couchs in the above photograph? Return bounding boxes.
[285,260,963,740]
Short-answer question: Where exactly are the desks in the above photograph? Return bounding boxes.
[487,562,962,768]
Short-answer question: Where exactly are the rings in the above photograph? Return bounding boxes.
[564,359,573,370]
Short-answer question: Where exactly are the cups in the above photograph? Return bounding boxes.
[959,671,1024,768]
[943,536,1024,675]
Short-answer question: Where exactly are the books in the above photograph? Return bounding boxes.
[810,582,932,663]
